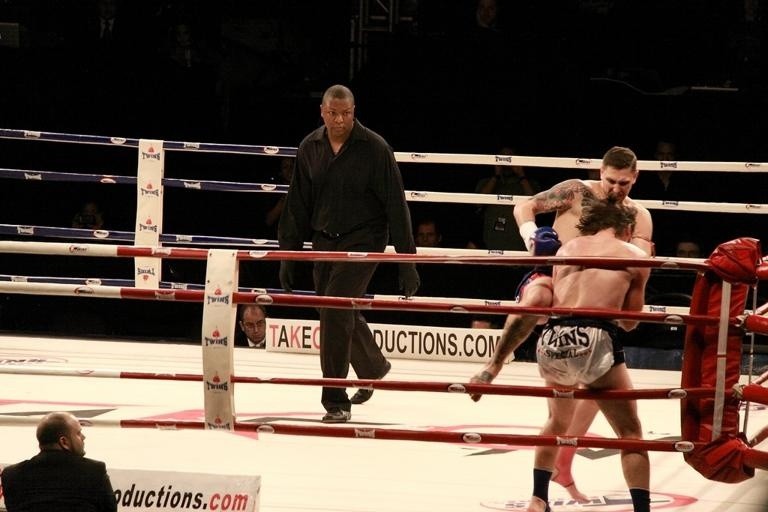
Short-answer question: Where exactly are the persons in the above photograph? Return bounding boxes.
[462,145,654,506]
[0,410,117,512]
[524,200,652,512]
[276,84,423,424]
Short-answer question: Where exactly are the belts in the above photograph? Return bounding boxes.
[319,229,354,242]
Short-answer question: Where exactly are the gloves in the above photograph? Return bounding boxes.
[522,225,562,256]
[279,259,302,292]
[397,262,421,295]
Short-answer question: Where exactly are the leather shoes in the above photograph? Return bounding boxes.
[350,361,391,405]
[321,409,351,423]
